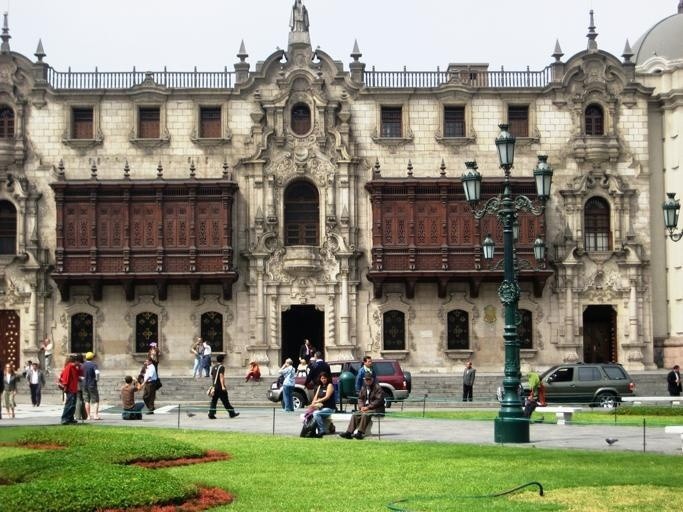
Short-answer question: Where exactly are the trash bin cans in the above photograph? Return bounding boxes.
[338,371,355,399]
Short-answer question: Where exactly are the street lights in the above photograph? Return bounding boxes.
[459,123,553,445]
[659,191,683,243]
[482,216,545,377]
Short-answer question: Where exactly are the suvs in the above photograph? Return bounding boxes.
[265,358,411,407]
[496,361,636,409]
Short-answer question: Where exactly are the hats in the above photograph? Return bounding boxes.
[363,372,374,379]
[85,352,95,359]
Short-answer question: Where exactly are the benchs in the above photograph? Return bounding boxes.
[521,407,584,425]
[664,426,683,449]
[300,413,385,436]
[621,396,683,407]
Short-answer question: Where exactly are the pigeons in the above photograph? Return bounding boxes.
[185,410,197,420]
[532,416,545,423]
[604,438,618,446]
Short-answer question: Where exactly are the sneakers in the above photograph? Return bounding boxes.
[316,432,326,438]
[208,413,215,418]
[231,412,239,417]
[339,432,352,439]
[354,433,362,439]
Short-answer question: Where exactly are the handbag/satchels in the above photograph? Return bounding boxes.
[206,386,215,396]
[300,419,317,438]
[152,378,162,391]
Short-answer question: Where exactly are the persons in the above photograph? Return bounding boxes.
[189,337,204,377]
[667,364,683,395]
[277,339,376,412]
[202,340,212,378]
[288,1,310,34]
[300,372,335,439]
[242,360,262,383]
[205,354,239,420]
[0,339,163,426]
[338,370,386,440]
[461,362,476,400]
